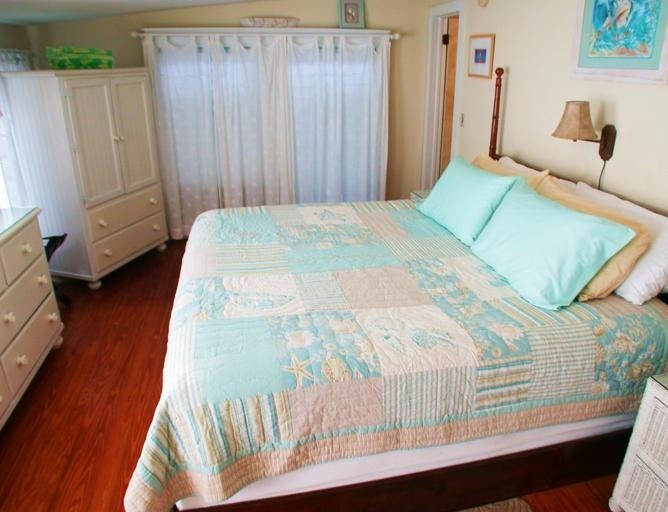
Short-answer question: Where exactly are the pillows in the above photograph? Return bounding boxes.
[417,152,668,310]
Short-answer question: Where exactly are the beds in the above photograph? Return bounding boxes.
[166,68,668,511]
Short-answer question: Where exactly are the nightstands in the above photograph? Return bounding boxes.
[608,373,668,511]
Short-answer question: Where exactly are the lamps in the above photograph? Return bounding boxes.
[552,101,617,160]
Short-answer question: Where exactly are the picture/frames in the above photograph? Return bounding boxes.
[571,0,668,82]
[467,34,495,79]
[338,0,365,29]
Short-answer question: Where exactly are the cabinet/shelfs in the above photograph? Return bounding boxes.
[0,206,65,430]
[0,67,171,291]
